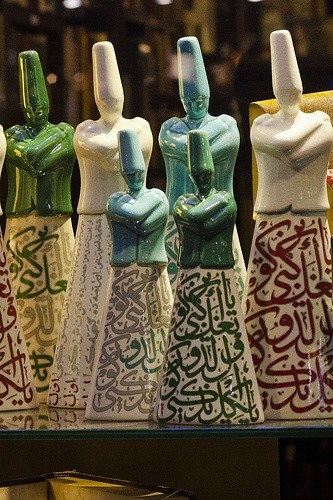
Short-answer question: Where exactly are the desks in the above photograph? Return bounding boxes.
[0,401,333,500]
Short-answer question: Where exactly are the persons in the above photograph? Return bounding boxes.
[240,28,333,421]
[0,49,76,408]
[45,40,153,413]
[158,35,249,311]
[85,131,176,421]
[0,126,38,409]
[147,129,268,426]
